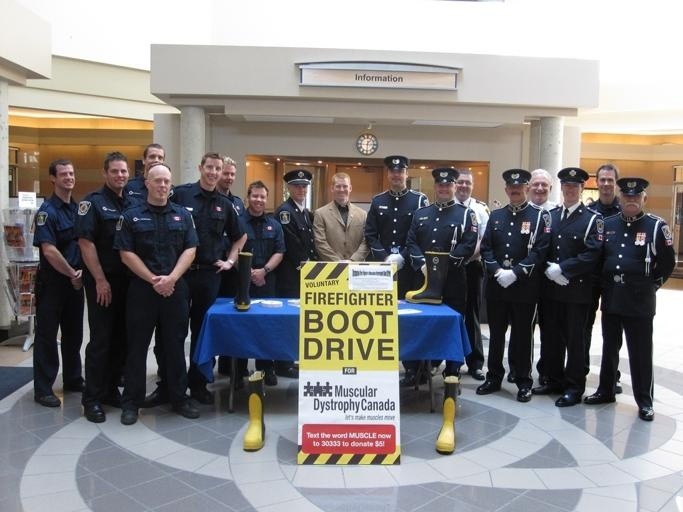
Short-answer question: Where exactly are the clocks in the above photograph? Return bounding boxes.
[355,133,378,155]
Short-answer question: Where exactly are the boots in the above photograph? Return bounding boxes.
[436,376,457,453]
[242,370,265,452]
[405,251,449,305]
[233,252,253,311]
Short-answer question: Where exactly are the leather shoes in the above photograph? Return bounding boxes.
[399,365,622,407]
[639,406,654,421]
[33,379,215,425]
[218,361,298,386]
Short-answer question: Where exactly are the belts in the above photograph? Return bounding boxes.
[187,262,217,271]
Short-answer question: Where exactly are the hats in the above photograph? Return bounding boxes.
[383,155,410,170]
[501,168,532,185]
[282,169,312,185]
[616,177,649,195]
[432,167,460,184]
[557,167,589,185]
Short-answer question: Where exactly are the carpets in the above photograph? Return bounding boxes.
[0,366,34,401]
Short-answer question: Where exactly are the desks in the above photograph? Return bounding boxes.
[191,296,472,414]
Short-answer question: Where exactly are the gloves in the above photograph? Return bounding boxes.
[544,261,562,280]
[384,253,405,271]
[493,267,517,288]
[544,263,569,286]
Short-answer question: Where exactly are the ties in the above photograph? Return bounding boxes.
[560,208,570,223]
